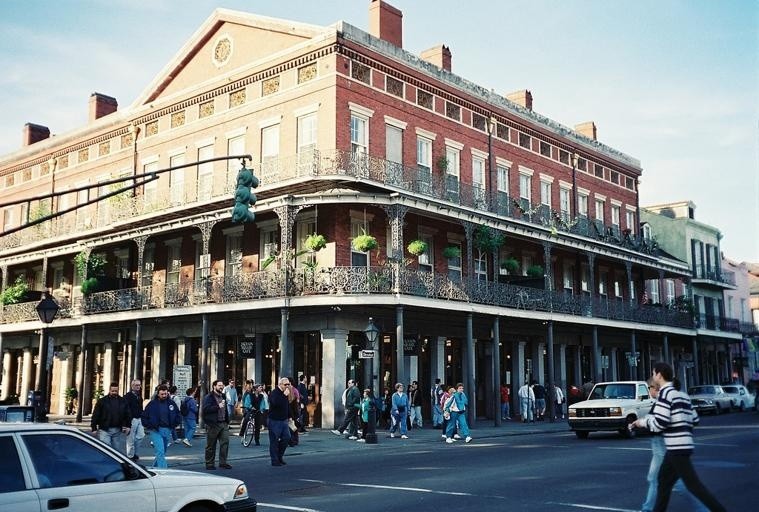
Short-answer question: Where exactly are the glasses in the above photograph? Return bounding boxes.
[284,384,291,386]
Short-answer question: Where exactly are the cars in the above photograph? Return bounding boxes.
[0,420,262,512]
[684,380,754,416]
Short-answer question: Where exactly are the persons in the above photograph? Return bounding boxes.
[477,378,595,423]
[631,360,729,512]
[91,375,309,470]
[331,379,422,442]
[629,375,668,512]
[430,378,472,443]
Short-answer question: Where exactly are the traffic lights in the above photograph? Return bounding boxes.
[245,169,261,229]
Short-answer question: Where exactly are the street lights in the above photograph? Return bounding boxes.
[364,316,381,413]
[35,289,60,421]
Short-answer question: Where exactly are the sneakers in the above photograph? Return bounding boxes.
[442,433,472,443]
[167,438,192,447]
[502,413,565,423]
[206,464,232,470]
[298,429,309,434]
[331,427,366,442]
[239,432,243,436]
[256,440,260,445]
[390,432,408,439]
[272,459,287,466]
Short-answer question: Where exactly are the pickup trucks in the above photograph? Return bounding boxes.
[565,379,657,437]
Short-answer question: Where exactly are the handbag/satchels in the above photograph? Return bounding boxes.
[398,405,405,413]
[444,396,455,409]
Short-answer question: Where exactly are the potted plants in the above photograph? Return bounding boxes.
[527,265,542,277]
[500,258,519,273]
[303,234,461,261]
